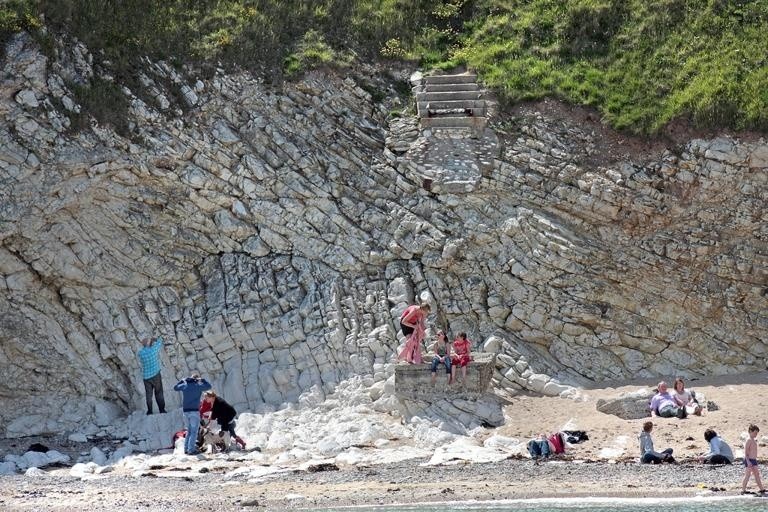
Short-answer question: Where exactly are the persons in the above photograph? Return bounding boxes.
[429,330,453,386]
[698,429,733,463]
[399,302,430,365]
[201,388,247,451]
[740,423,767,494]
[649,380,688,419]
[525,433,557,463]
[640,421,674,464]
[449,331,472,384]
[137,337,166,416]
[174,373,212,456]
[672,377,708,417]
